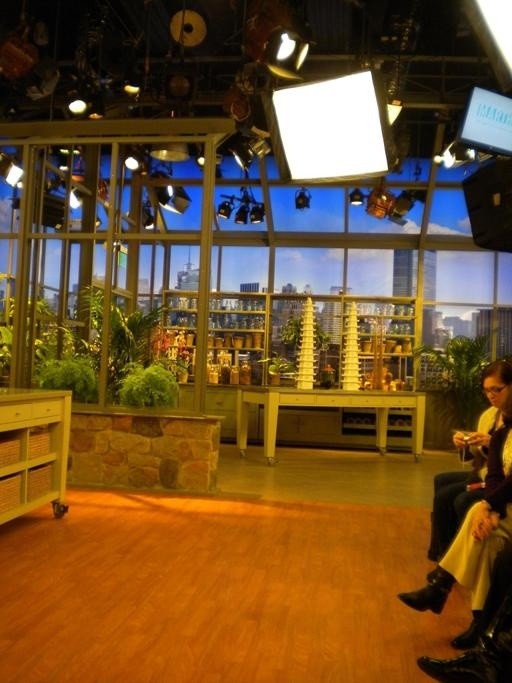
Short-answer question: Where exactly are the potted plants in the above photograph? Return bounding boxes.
[408,326,502,450]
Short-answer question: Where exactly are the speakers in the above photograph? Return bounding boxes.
[462,159,511,253]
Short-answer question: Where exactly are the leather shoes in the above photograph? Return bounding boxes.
[417,636,512,683]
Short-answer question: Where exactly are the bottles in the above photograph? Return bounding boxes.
[186,330,194,346]
[319,364,336,389]
[208,298,264,329]
[207,350,252,384]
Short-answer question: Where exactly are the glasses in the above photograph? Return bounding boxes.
[484,384,507,392]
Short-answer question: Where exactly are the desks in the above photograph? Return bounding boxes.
[236,385,427,466]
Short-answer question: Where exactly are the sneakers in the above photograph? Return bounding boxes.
[427,566,457,585]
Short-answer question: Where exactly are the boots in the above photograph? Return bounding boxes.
[397,567,454,614]
[451,610,491,650]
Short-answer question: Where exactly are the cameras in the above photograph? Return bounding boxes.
[463,432,473,444]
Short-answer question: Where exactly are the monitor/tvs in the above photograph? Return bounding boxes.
[457,85,511,155]
[262,69,402,184]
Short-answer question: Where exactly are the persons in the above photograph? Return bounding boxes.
[398,393,512,647]
[427,358,512,559]
[418,589,512,683]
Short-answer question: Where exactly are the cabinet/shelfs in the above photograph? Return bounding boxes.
[157,288,423,452]
[0,387,73,526]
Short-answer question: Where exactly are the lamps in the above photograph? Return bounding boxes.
[0,0,475,231]
